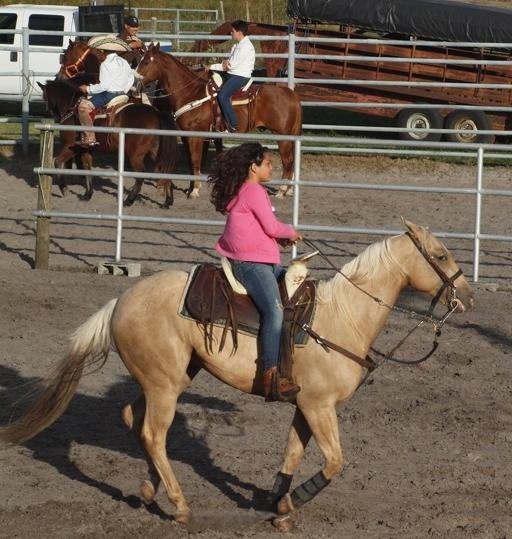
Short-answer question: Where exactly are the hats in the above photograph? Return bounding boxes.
[125,16,138,27]
[88,34,131,52]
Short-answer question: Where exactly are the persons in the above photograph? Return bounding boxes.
[207,141,304,403]
[204,20,256,134]
[76,33,135,145]
[115,15,147,69]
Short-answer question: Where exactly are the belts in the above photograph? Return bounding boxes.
[109,91,125,94]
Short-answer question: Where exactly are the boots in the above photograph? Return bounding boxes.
[264,367,300,401]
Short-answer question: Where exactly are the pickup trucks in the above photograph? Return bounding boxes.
[0,4,173,106]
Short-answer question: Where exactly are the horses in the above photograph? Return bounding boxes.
[135,41,306,199]
[35,73,178,213]
[55,36,135,86]
[0,212,476,534]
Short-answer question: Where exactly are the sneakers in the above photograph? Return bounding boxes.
[220,122,239,130]
